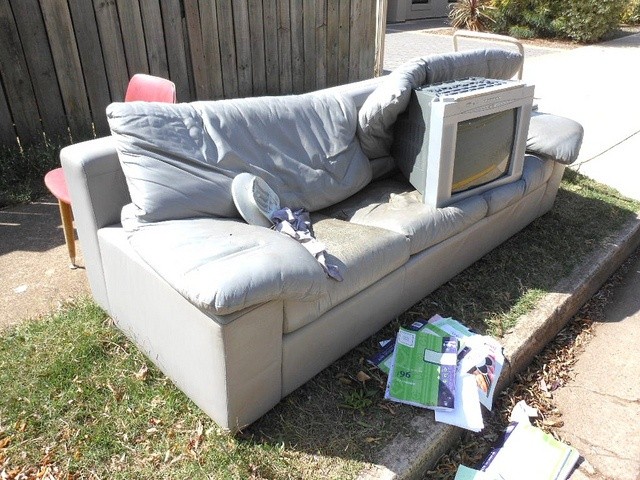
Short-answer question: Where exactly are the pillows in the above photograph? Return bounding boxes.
[105,94,373,221]
[120,203,328,315]
[358,47,525,159]
[527,111,584,164]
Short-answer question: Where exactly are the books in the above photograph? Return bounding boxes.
[454,420,580,480]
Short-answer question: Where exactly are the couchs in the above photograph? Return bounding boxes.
[56,41,586,437]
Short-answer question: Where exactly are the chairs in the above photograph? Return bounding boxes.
[42,69,178,272]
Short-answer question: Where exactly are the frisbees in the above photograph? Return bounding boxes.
[231,172,273,227]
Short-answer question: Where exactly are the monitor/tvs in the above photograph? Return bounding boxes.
[392,75,535,208]
